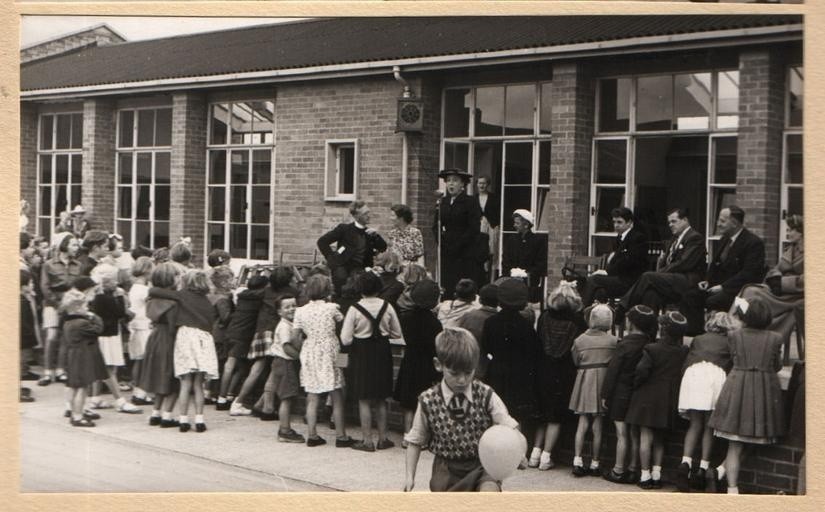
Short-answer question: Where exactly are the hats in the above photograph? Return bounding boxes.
[439,167,472,180]
[499,278,529,310]
[53,232,73,251]
[624,305,655,328]
[513,209,534,226]
[71,205,85,215]
[410,280,439,311]
[657,312,687,335]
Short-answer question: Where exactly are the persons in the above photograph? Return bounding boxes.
[21,166,804,494]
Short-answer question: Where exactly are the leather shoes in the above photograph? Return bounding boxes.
[206,396,279,420]
[279,429,326,445]
[119,395,155,412]
[149,416,207,433]
[674,463,718,493]
[65,400,113,426]
[22,371,67,385]
[336,436,394,451]
[528,459,554,470]
[573,465,662,488]
